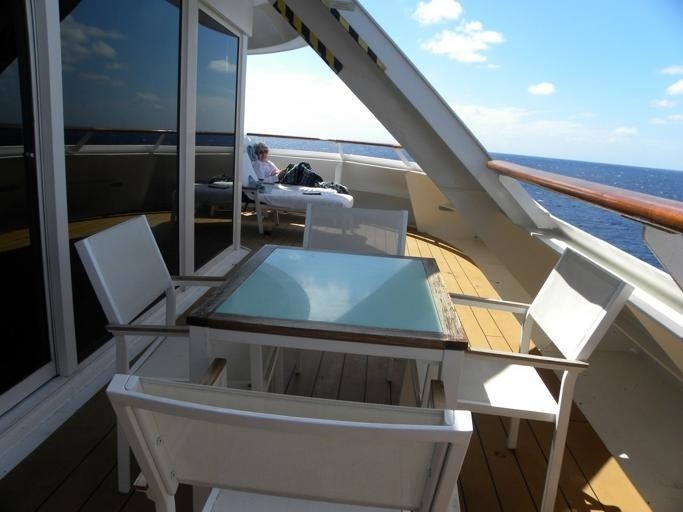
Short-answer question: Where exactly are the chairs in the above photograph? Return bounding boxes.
[106,374,474,512]
[74,212,225,497]
[448,244,636,511]
[243,134,354,235]
[300,202,409,256]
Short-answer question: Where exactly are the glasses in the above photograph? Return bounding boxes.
[259,149,268,154]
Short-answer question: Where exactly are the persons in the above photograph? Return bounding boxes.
[251,142,325,187]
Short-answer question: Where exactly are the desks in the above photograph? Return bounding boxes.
[174,242,469,413]
